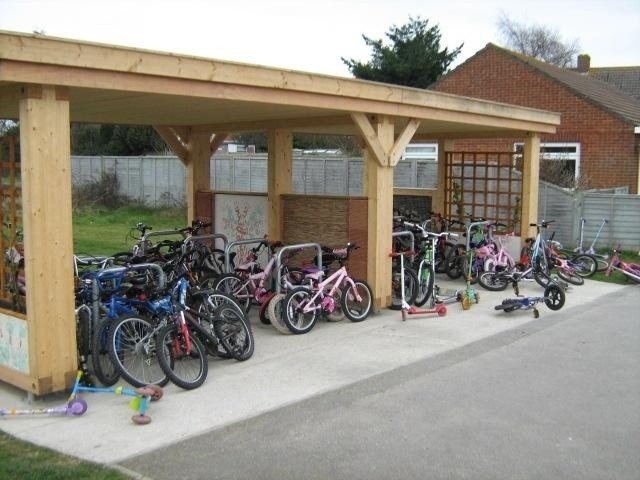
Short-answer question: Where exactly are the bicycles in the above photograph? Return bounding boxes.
[6,218,374,395]
[391,203,640,320]
